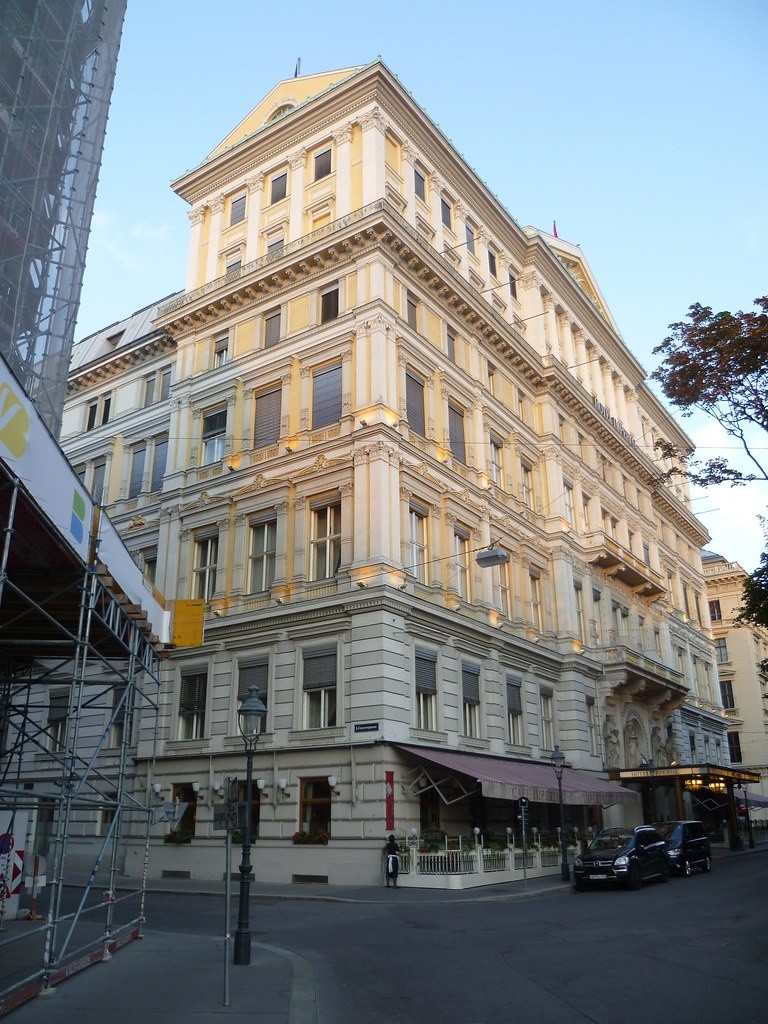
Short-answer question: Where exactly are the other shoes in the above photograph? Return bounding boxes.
[386,885,391,888]
[394,886,400,889]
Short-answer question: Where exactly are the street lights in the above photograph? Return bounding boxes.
[550,744,570,881]
[234,687,270,966]
[742,783,754,849]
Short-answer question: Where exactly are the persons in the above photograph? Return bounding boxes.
[626,721,641,769]
[651,727,681,767]
[604,714,620,768]
[383,835,401,889]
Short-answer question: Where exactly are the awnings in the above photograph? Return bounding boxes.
[385,738,638,806]
[700,785,768,809]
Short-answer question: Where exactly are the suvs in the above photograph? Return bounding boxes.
[574,824,670,886]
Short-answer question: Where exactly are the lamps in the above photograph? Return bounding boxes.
[578,650,585,655]
[498,622,504,627]
[533,638,540,642]
[409,827,594,849]
[213,610,222,616]
[278,778,290,797]
[356,580,367,588]
[275,597,285,604]
[686,698,729,719]
[454,605,462,612]
[683,775,727,795]
[192,782,204,800]
[671,761,676,765]
[328,776,340,795]
[398,582,408,590]
[476,541,510,569]
[256,779,269,797]
[153,784,165,800]
[214,781,224,798]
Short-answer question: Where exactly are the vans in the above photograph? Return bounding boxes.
[651,821,713,878]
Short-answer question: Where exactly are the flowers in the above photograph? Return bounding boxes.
[293,829,329,845]
